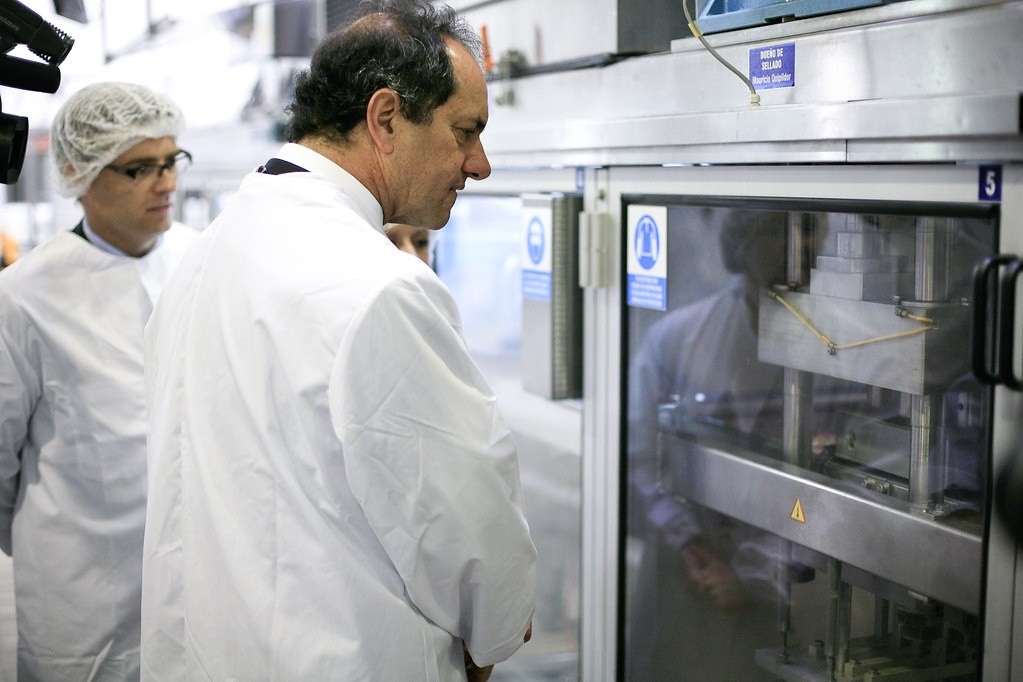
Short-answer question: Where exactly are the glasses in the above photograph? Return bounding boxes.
[106,149,192,185]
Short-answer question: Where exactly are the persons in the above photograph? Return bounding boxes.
[383,222,434,268]
[139,0,539,682]
[0,80,199,682]
[627,204,826,682]
